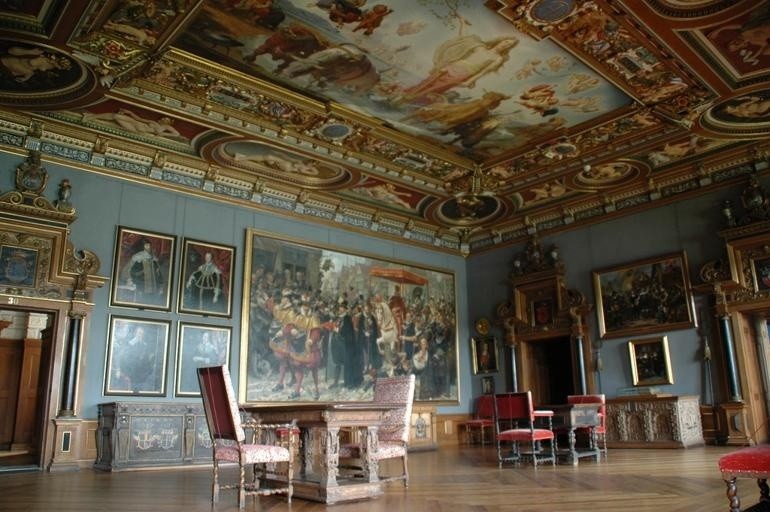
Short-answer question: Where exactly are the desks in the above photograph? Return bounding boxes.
[238,403,407,504]
[605,394,706,449]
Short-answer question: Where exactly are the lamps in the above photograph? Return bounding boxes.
[594,349,604,394]
[703,334,715,407]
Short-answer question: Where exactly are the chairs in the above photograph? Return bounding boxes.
[459,391,606,470]
[719,447,769,512]
[196,366,295,512]
[339,374,415,487]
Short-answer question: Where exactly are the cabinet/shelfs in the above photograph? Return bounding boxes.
[94,402,240,472]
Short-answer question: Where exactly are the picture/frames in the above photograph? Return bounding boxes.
[102,313,172,396]
[239,226,462,410]
[591,249,699,339]
[470,337,501,395]
[749,252,770,293]
[627,335,673,387]
[108,225,178,312]
[174,321,234,398]
[178,238,237,319]
